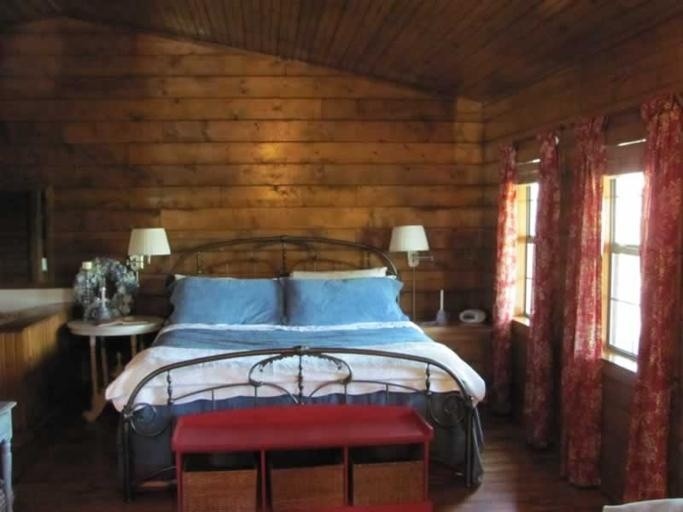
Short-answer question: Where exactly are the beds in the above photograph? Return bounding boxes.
[116,236,474,498]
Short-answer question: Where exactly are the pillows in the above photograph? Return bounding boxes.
[172,273,409,327]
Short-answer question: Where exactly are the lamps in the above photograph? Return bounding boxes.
[386,224,431,326]
[126,227,171,313]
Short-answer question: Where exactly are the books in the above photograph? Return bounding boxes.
[95,315,151,327]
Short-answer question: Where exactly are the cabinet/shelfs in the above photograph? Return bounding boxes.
[172,404,433,512]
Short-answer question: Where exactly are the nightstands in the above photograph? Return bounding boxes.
[422,327,497,387]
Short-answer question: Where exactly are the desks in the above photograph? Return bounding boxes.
[66,314,164,403]
[0,399,18,512]
